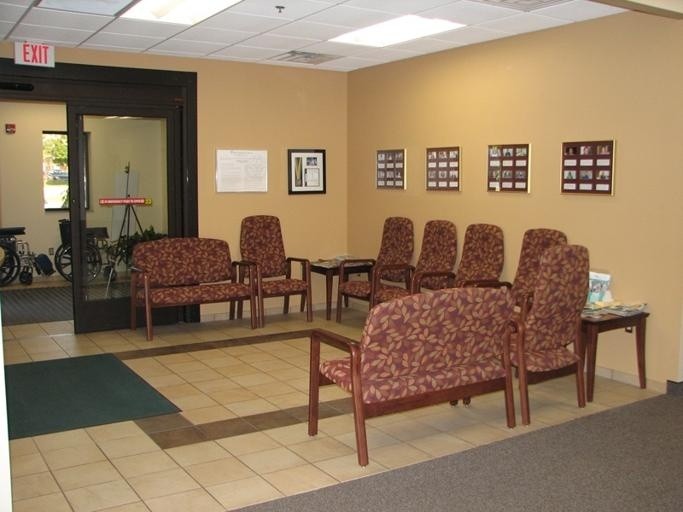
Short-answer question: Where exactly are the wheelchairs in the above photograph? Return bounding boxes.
[0,218,119,288]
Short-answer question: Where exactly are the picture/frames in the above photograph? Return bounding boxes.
[287,148,326,196]
[375,138,617,197]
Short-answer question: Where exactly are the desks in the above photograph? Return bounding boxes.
[301,257,373,320]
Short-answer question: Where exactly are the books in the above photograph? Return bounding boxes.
[578,270,652,324]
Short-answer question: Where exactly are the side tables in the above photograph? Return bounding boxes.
[576,304,650,401]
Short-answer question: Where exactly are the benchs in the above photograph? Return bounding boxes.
[307,285,518,468]
[127,235,258,343]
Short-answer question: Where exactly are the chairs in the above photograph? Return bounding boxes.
[462,244,589,425]
[233,215,314,330]
[336,216,570,324]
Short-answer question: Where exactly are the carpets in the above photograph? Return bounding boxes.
[3,352,182,441]
[224,394,682,512]
[0,283,132,327]
